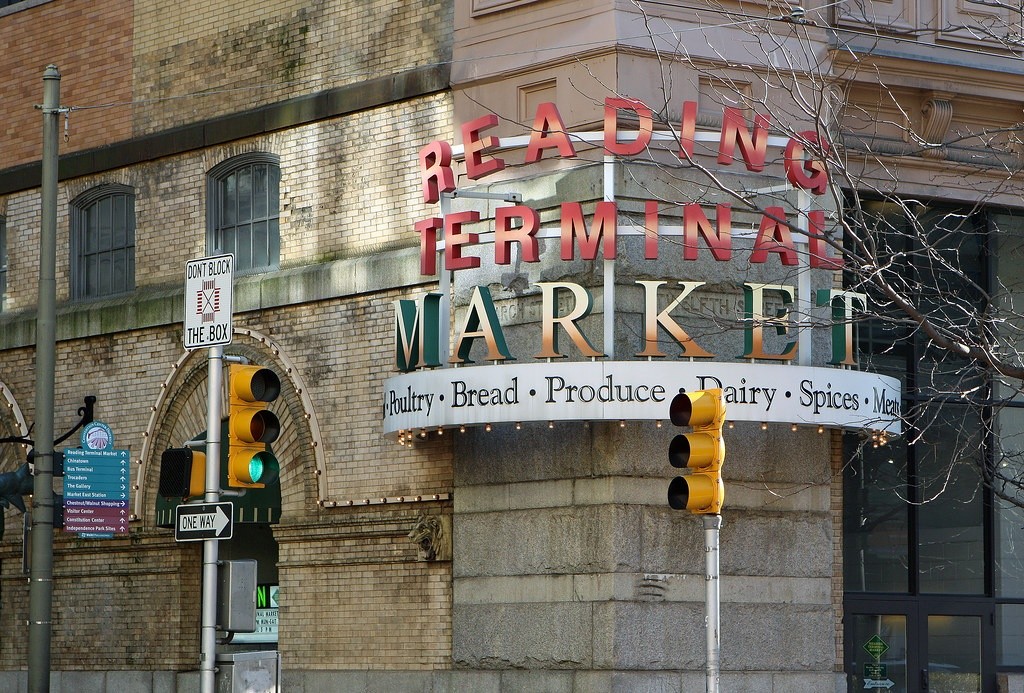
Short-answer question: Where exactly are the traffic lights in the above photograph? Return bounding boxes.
[225,364,280,488]
[159,445,206,498]
[668,389,722,515]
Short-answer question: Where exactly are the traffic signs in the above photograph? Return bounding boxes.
[174,502,234,542]
[62,446,128,539]
